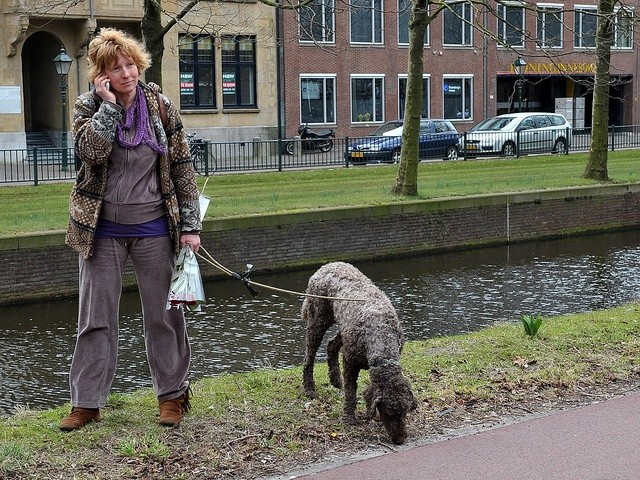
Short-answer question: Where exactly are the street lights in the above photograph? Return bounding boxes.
[512,54,528,111]
[49,44,76,172]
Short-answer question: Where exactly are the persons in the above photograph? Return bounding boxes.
[456,109,462,119]
[364,109,371,121]
[357,112,364,122]
[58,26,203,432]
[465,108,470,119]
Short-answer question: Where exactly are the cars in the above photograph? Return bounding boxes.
[454,116,495,155]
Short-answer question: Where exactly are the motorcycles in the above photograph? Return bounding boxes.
[282,122,338,156]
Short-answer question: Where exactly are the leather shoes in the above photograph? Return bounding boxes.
[58,407,101,431]
[159,398,183,427]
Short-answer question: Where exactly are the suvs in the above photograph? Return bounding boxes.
[343,118,462,166]
[459,111,574,158]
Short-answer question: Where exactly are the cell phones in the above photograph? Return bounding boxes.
[106,81,112,92]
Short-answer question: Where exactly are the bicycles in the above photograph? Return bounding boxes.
[185,132,218,177]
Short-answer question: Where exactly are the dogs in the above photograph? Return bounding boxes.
[300,261,418,445]
